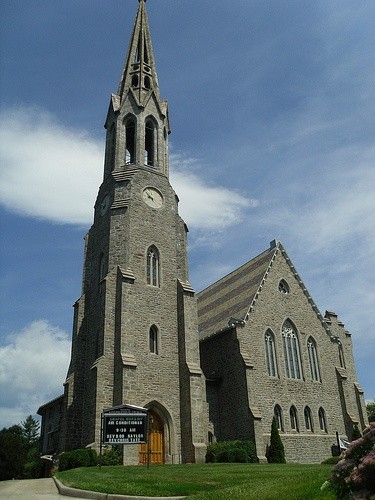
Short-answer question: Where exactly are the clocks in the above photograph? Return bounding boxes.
[143,186,165,208]
